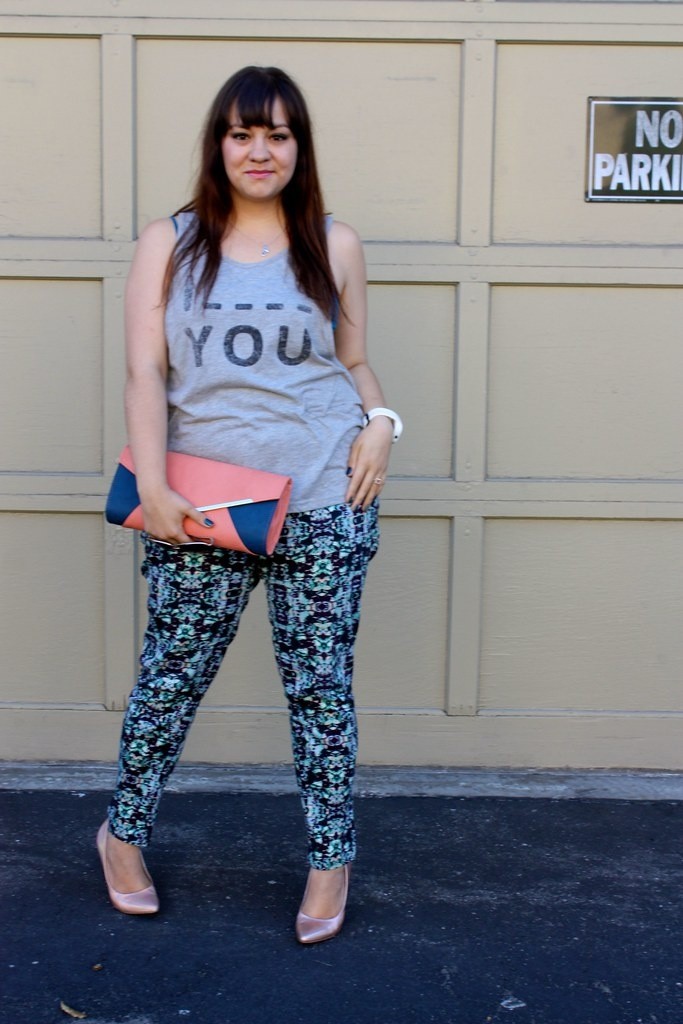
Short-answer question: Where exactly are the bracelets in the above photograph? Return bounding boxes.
[363,405,403,443]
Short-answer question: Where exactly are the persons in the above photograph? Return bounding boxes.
[96,67,394,943]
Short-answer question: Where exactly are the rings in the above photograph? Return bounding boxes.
[374,478,383,485]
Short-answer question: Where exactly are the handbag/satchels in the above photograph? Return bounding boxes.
[104,444,292,557]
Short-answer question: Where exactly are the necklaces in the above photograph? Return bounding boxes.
[227,221,288,257]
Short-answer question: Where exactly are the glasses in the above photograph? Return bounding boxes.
[139,531,215,553]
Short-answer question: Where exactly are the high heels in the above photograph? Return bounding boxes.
[296,862,349,943]
[96,819,160,914]
